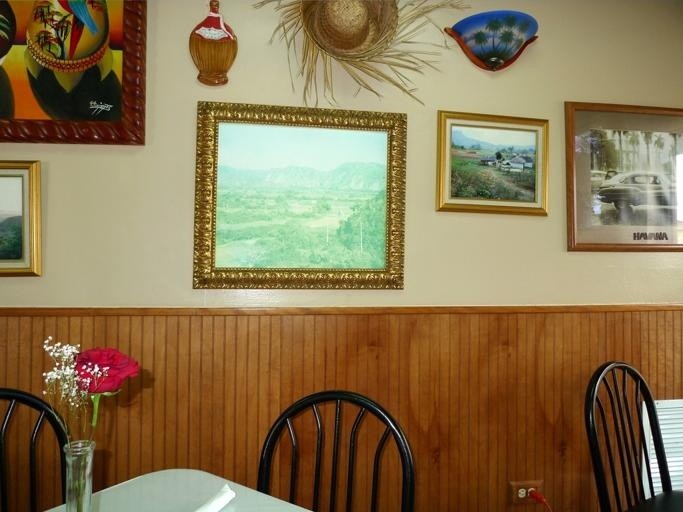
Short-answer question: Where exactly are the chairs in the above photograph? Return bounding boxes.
[0,388,69,510]
[583,358,682,510]
[256,388,414,512]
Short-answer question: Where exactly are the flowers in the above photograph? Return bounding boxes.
[36,334,142,511]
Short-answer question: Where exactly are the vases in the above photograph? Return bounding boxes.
[60,439,94,512]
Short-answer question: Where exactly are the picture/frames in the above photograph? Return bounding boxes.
[561,100,683,252]
[0,0,148,146]
[190,98,408,291]
[0,161,40,278]
[435,110,551,218]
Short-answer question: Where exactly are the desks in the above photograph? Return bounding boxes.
[43,468,313,512]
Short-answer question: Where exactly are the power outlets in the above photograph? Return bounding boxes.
[508,479,545,505]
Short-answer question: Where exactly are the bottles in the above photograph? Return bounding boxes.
[188,0,238,86]
